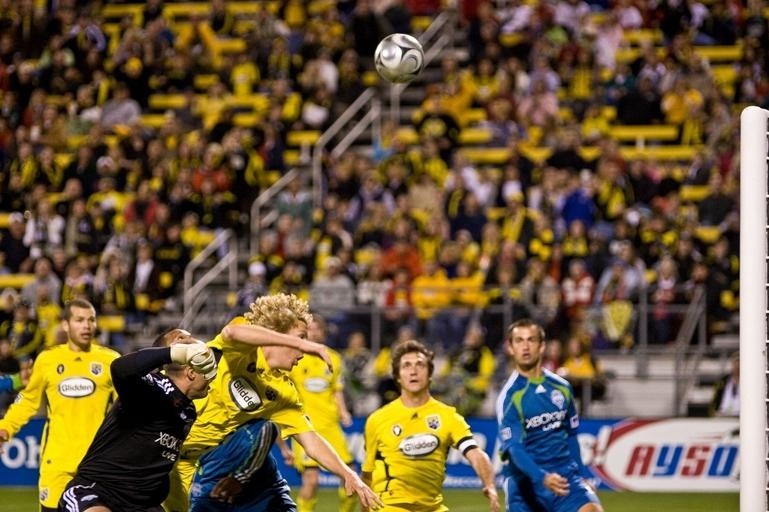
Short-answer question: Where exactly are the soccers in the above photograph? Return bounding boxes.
[374,33,425,84]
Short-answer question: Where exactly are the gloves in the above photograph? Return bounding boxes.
[170,343,216,374]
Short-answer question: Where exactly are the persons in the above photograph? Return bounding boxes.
[159,291,385,512]
[56,342,215,512]
[361,340,500,512]
[495,318,604,512]
[0,298,123,512]
[292,314,360,512]
[150,329,298,512]
[274,433,295,466]
